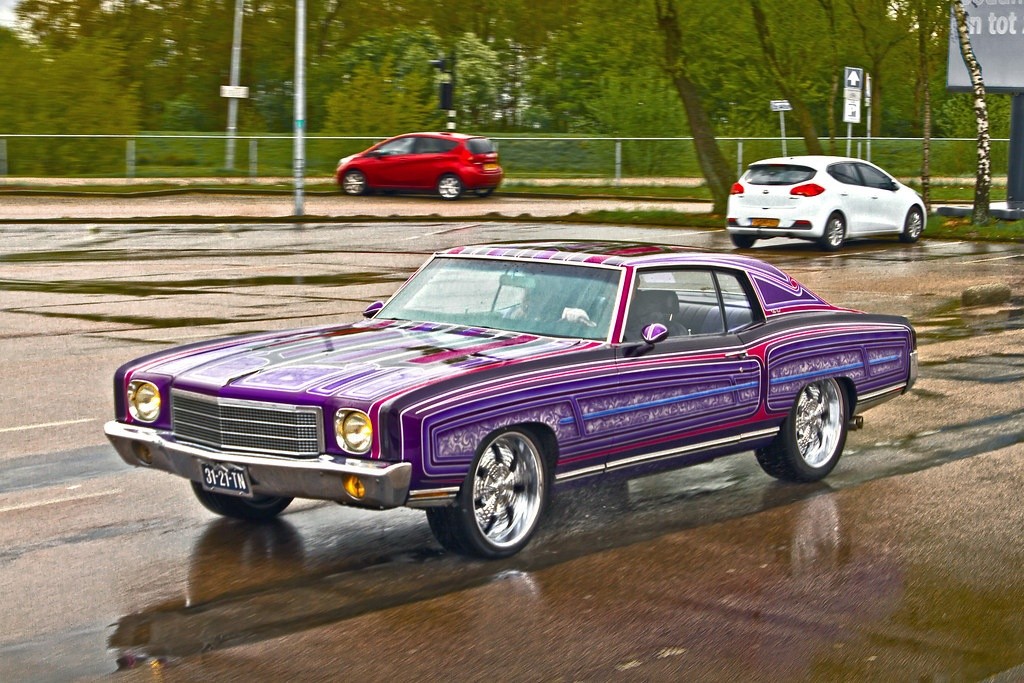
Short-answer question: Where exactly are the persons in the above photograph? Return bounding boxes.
[502,274,561,326]
[561,272,668,341]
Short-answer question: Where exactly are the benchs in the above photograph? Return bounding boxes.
[674,301,751,335]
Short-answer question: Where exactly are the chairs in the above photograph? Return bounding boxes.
[644,289,687,337]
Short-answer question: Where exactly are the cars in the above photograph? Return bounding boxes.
[725,154,928,259]
[103,239,925,560]
[334,129,505,203]
